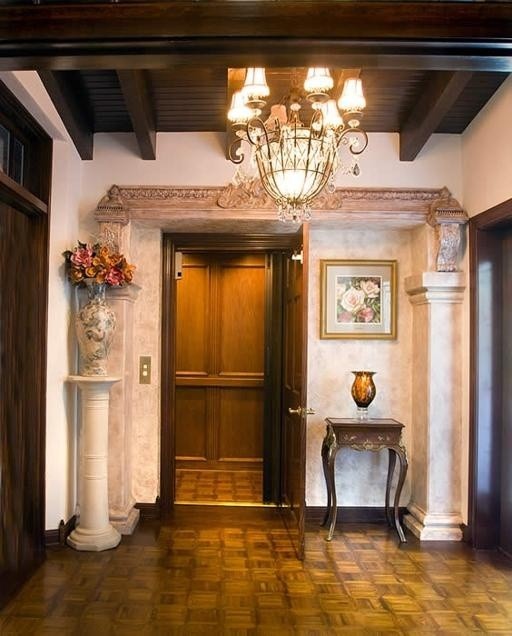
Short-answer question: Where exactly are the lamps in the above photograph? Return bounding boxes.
[224,66,370,225]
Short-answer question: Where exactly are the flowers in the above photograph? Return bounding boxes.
[61,238,137,289]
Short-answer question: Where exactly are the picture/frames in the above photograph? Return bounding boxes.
[319,258,399,340]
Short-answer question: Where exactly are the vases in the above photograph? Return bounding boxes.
[351,369,379,421]
[72,277,117,377]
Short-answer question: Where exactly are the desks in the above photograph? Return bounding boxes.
[319,416,411,543]
